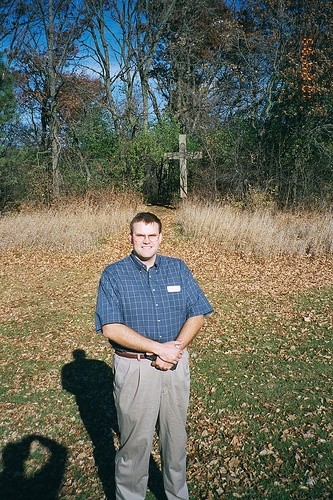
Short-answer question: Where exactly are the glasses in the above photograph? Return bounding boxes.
[131,233,161,242]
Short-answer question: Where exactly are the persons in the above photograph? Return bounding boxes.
[96,212,214,499]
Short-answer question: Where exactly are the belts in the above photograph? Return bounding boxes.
[115,349,155,360]
